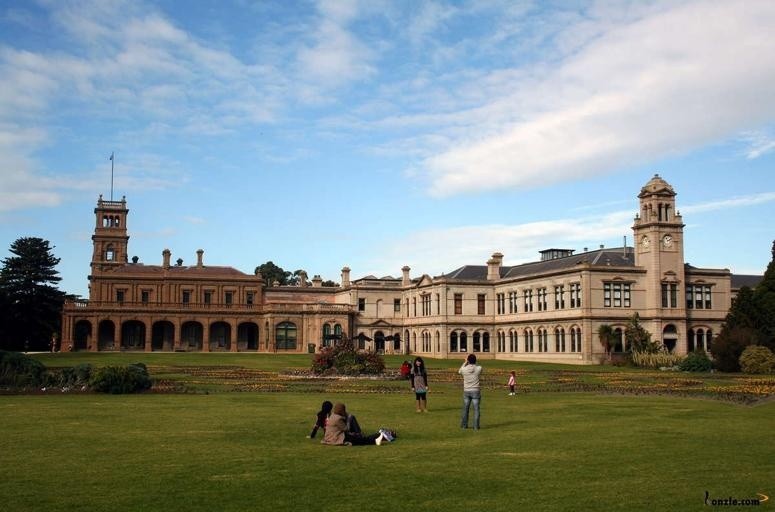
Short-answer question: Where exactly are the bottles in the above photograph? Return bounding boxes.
[334,402,346,415]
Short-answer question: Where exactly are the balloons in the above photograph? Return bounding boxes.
[642,237,649,247]
[662,235,673,247]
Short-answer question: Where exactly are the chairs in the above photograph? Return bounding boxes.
[460,425,469,429]
[375,432,384,446]
[472,426,481,432]
[379,427,395,442]
[508,392,512,396]
[513,392,516,395]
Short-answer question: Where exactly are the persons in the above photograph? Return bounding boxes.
[320,403,384,446]
[410,356,428,414]
[457,354,482,430]
[306,400,333,438]
[401,360,412,376]
[507,370,517,397]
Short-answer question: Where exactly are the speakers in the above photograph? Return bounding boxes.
[422,399,428,413]
[416,399,422,413]
